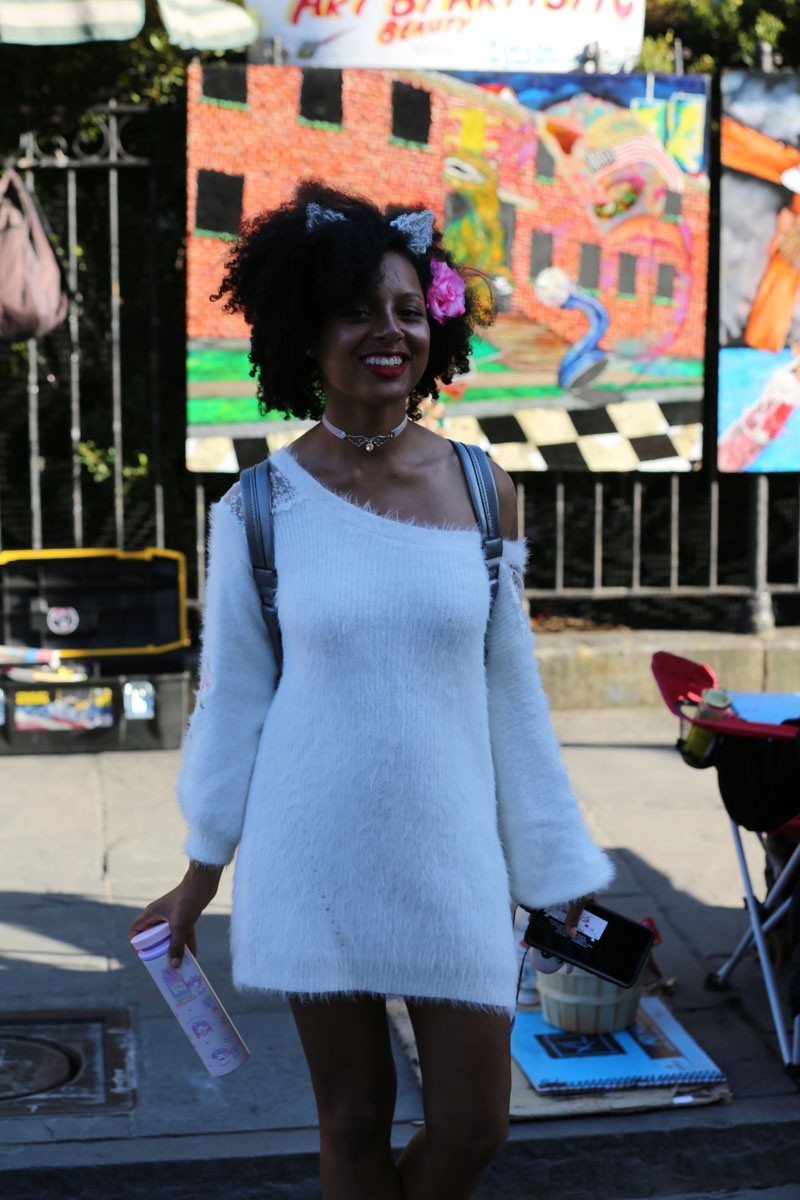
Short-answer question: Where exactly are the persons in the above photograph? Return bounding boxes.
[127,173,614,1199]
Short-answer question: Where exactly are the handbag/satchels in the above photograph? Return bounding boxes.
[0,150,68,343]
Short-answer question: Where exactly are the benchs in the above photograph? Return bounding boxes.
[0,548,204,746]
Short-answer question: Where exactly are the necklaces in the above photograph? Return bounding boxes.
[322,412,408,452]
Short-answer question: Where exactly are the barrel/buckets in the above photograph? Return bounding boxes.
[530,964,644,1034]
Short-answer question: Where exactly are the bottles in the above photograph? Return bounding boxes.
[130,921,249,1077]
[684,687,731,757]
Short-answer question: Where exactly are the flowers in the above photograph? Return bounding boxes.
[424,258,468,326]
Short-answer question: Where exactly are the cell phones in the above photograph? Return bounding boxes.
[524,897,655,989]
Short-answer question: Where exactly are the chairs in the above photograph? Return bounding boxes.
[648,648,799,1068]
